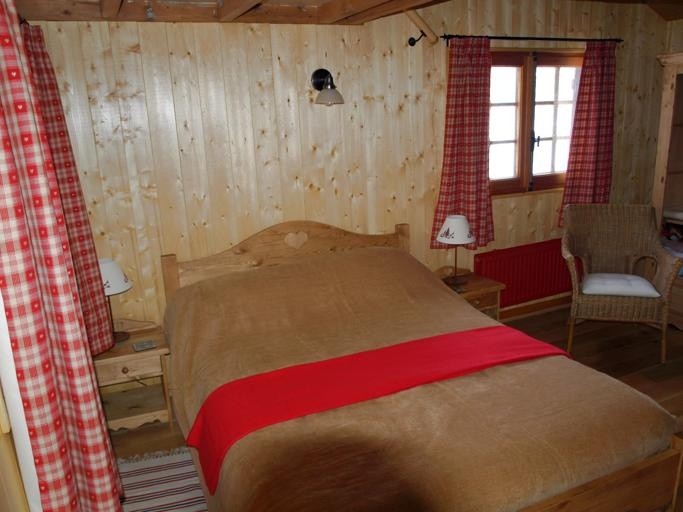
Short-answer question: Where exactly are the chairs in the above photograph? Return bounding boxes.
[560,199,683,363]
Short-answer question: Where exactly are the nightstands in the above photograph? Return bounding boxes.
[90,326,173,436]
[437,272,506,321]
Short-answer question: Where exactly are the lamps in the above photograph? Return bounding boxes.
[311,68,344,106]
[436,215,475,270]
[100,260,135,339]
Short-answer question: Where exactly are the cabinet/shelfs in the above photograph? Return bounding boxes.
[653,49,683,331]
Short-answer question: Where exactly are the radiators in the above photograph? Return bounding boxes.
[472,235,571,306]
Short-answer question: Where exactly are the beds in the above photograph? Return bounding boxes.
[157,221,683,512]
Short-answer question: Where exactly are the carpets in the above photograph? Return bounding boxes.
[110,449,207,512]
